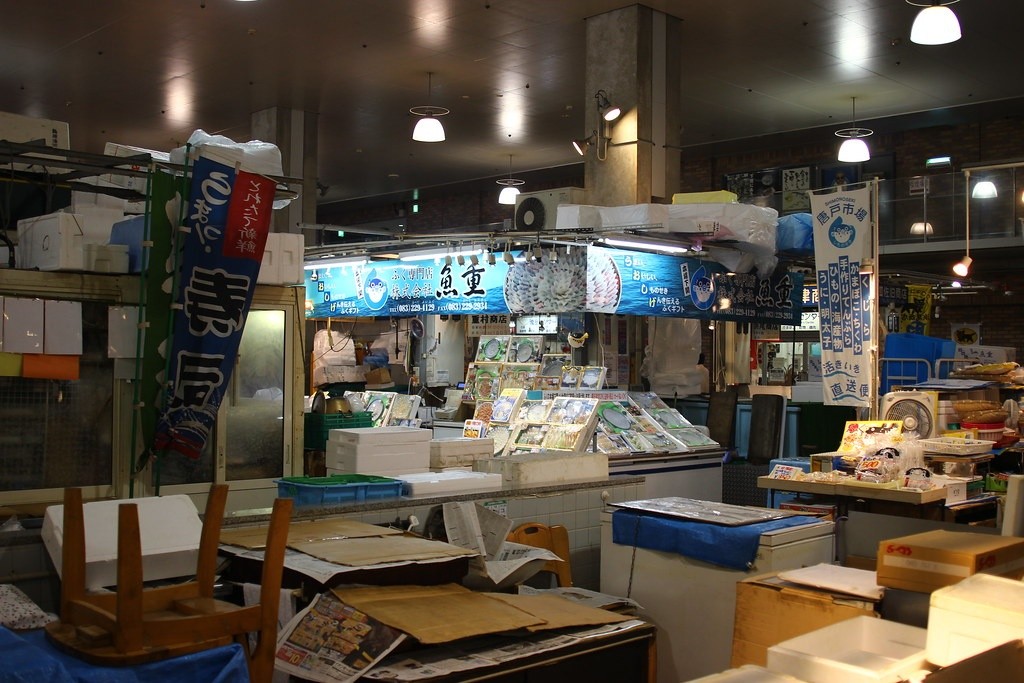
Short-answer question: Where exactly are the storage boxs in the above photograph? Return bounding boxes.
[555,190,817,254]
[0,110,307,288]
[39,493,213,590]
[273,335,721,513]
[684,528,1024,683]
[756,334,1024,527]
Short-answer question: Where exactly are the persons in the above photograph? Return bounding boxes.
[828,169,853,193]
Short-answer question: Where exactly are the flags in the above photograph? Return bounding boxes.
[807,182,873,408]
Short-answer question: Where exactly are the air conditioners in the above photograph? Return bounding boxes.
[514,187,583,230]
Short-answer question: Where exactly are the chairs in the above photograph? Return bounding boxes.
[43,485,294,683]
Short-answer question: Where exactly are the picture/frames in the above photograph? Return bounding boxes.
[751,166,781,214]
[816,159,863,195]
[724,172,754,195]
[780,163,814,214]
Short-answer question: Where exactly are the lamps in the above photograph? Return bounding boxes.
[910,221,934,235]
[834,96,874,164]
[971,178,999,198]
[410,71,450,142]
[572,89,621,161]
[906,0,963,46]
[953,170,973,276]
[496,155,527,205]
